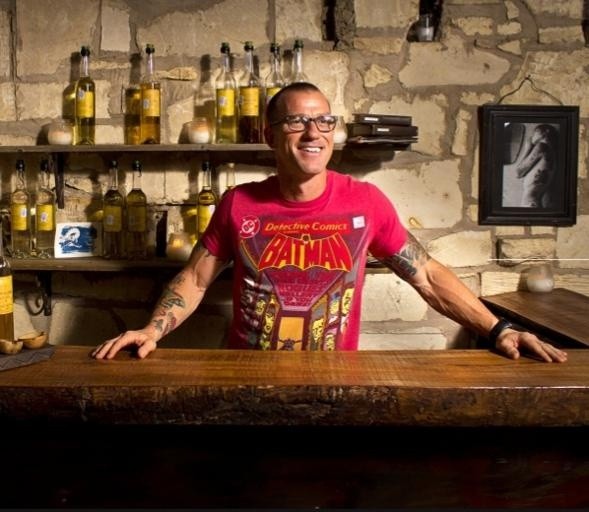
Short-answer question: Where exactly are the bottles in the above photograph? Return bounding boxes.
[215,39,310,144]
[193,49,293,118]
[11,158,236,259]
[73,45,96,146]
[0,216,14,342]
[140,42,161,144]
[124,54,141,145]
[86,168,220,247]
[62,52,81,127]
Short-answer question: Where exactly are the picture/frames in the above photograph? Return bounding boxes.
[478,104,579,226]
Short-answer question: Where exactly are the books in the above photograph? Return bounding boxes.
[346,112,418,138]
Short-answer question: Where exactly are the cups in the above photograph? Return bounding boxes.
[416,13,434,41]
[187,116,211,143]
[48,119,76,145]
[527,263,555,293]
[332,116,348,143]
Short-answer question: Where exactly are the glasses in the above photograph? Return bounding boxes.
[271,115,337,132]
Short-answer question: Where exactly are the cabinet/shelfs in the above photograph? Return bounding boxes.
[0,144,393,272]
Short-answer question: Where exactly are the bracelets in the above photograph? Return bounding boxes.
[486,316,513,356]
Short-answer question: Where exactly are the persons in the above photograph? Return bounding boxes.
[515,125,556,207]
[90,82,570,364]
